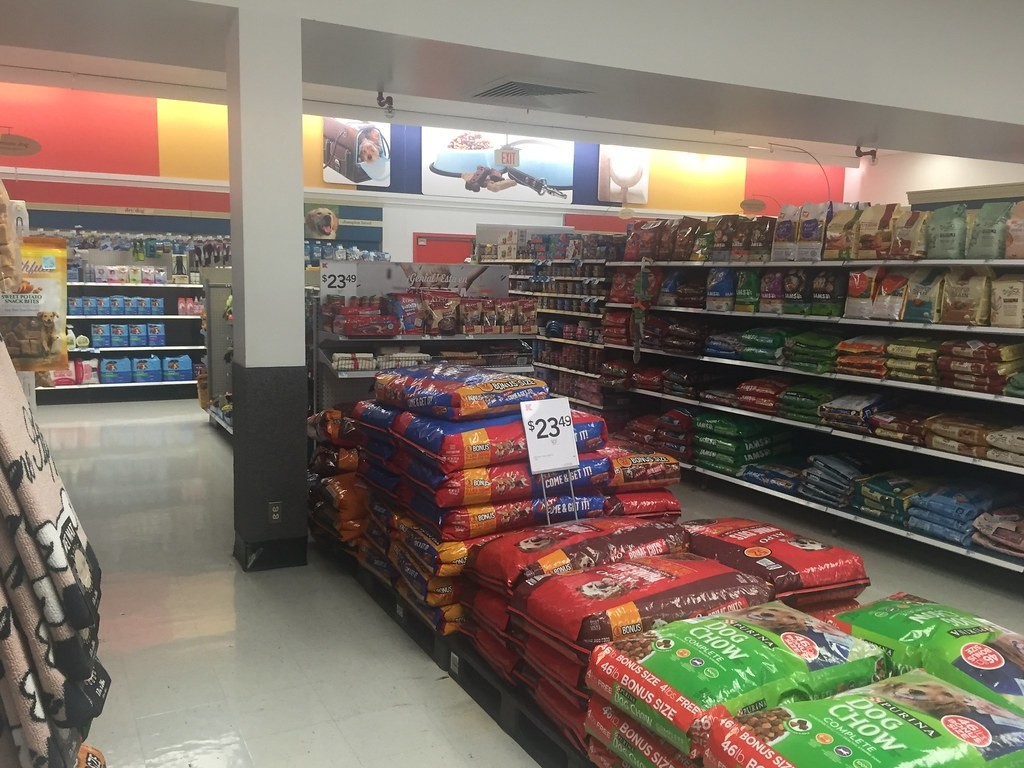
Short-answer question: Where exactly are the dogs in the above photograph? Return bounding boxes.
[871,680,971,718]
[304,207,338,239]
[36,310,60,359]
[720,605,810,633]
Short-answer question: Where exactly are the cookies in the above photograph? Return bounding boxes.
[460,167,517,192]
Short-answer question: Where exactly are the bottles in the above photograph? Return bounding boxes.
[36,226,231,269]
[304,240,391,270]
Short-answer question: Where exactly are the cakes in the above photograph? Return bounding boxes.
[432,132,506,174]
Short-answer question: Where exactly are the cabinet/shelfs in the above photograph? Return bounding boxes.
[37,254,1024,574]
